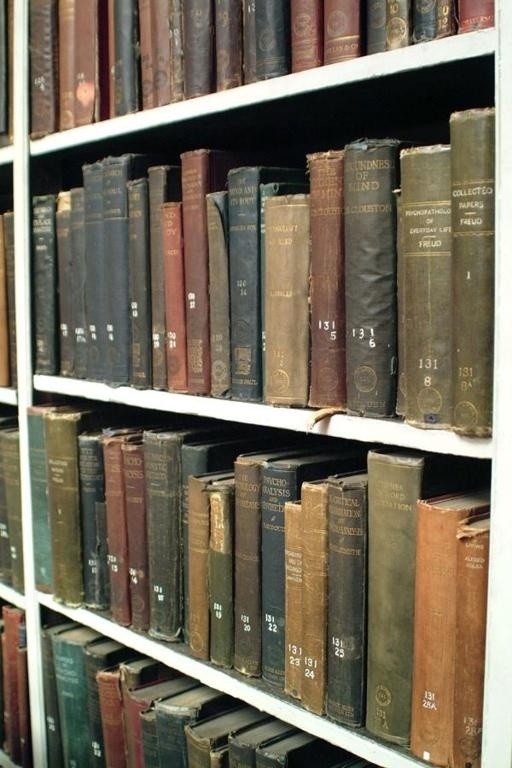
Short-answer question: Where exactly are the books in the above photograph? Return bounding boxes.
[0,3,496,147]
[2,406,489,768]
[1,110,496,433]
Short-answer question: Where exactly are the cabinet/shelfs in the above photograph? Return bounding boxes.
[0,0,509,768]
